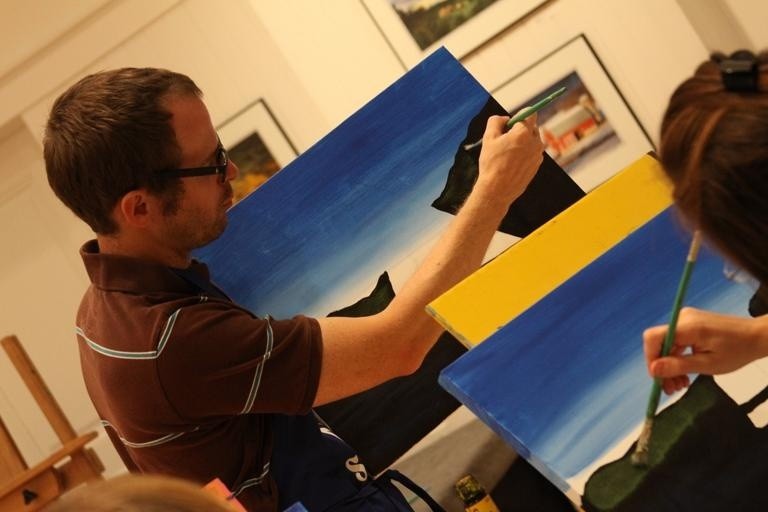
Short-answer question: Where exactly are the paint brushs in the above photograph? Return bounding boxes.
[631,230,703,466]
[464,87,568,150]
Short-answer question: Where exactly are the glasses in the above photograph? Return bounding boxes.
[140,142,229,182]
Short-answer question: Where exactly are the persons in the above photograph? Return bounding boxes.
[44,67,546,512]
[643,49,767,395]
